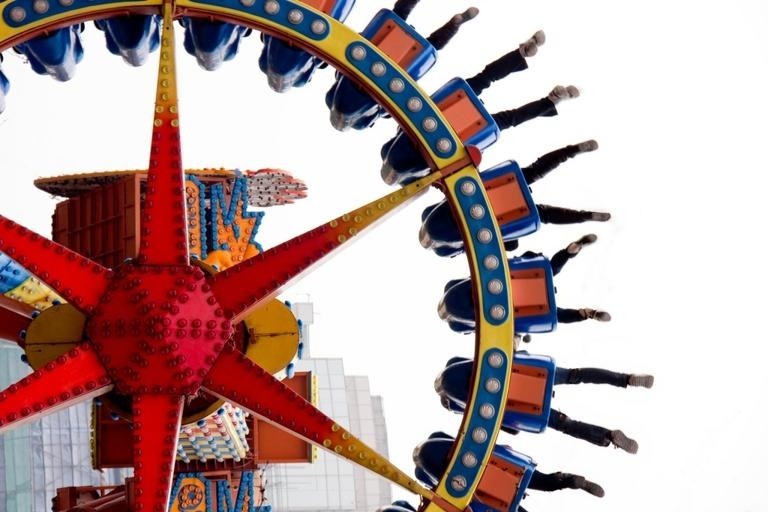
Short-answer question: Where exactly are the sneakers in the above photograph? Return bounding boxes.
[580,308,611,322]
[453,7,479,24]
[629,376,654,389]
[519,30,546,57]
[580,140,599,152]
[550,86,581,101]
[572,476,605,498]
[591,212,611,222]
[568,234,597,254]
[611,430,639,455]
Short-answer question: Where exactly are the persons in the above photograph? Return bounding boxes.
[392,0,480,53]
[465,28,581,134]
[520,139,612,226]
[516,468,604,512]
[550,233,611,325]
[545,368,654,455]
